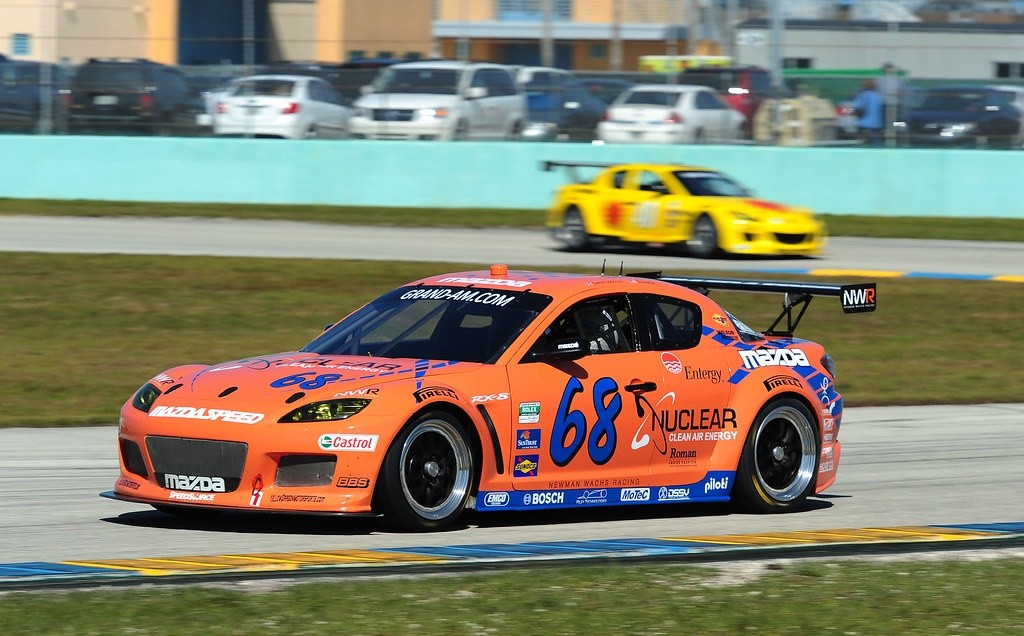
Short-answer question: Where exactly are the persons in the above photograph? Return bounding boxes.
[850,77,887,146]
[555,306,619,354]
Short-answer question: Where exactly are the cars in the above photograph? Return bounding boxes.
[892,83,1024,150]
[0,52,777,145]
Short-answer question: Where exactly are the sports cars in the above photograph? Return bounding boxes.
[97,260,876,532]
[540,157,829,262]
